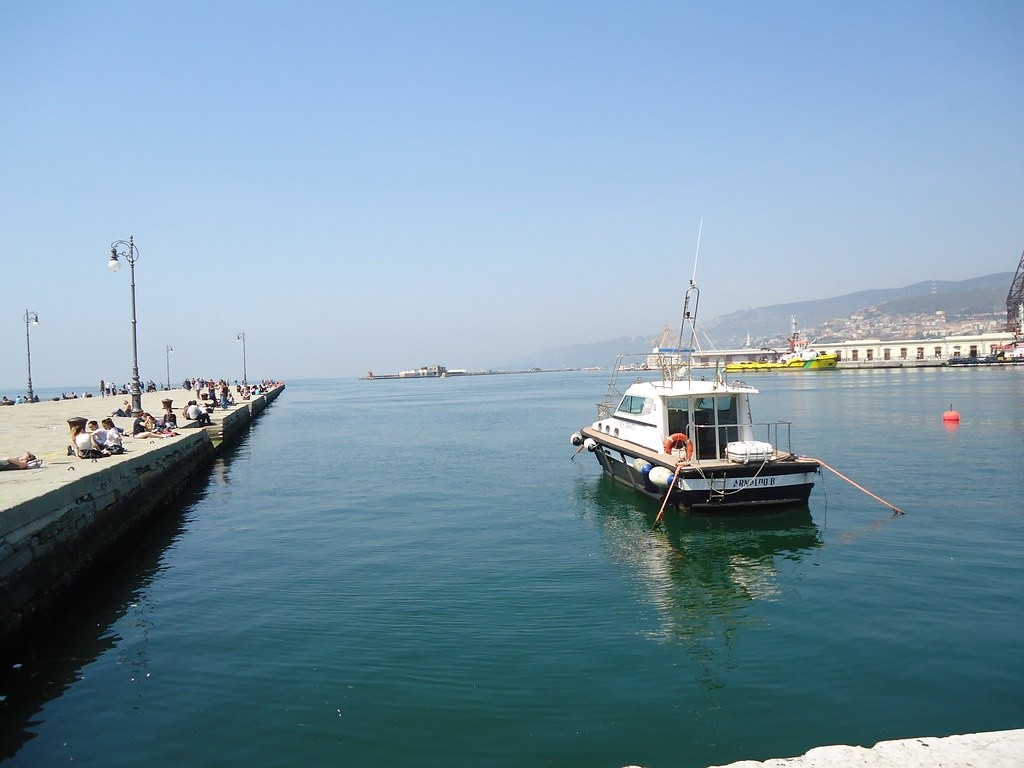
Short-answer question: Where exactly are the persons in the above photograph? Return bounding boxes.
[34,395,40,402]
[195,379,202,399]
[133,413,181,438]
[218,379,223,390]
[88,421,106,443]
[220,382,228,408]
[61,374,168,398]
[182,377,281,400]
[108,400,131,417]
[187,400,211,426]
[210,386,218,407]
[15,396,22,404]
[164,408,177,428]
[0,452,36,471]
[227,392,234,403]
[94,418,123,454]
[183,401,192,420]
[68,425,93,458]
[209,379,214,386]
[24,396,28,403]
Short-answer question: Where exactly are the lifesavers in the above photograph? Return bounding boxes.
[663,432,694,461]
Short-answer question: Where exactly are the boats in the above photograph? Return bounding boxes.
[569,217,822,514]
[724,313,839,373]
[946,353,1024,367]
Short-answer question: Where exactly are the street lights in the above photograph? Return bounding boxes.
[166,344,174,390]
[236,330,247,389]
[108,234,143,418]
[23,309,39,403]
